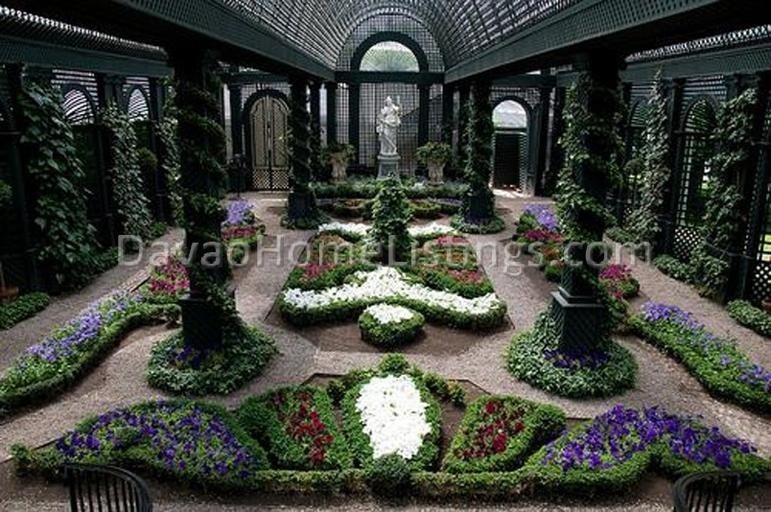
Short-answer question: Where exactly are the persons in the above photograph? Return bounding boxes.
[375,96,402,156]
